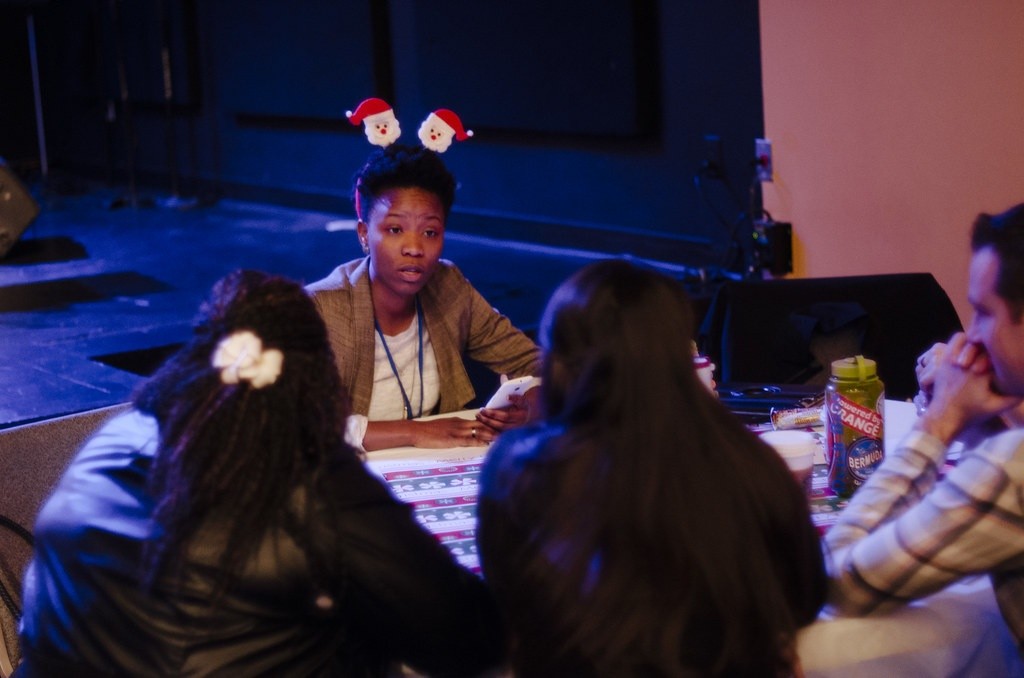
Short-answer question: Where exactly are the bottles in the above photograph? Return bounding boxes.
[825,357,886,497]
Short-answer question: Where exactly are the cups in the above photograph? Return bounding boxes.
[696,356,715,393]
[758,430,815,515]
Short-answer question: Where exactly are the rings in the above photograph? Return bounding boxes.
[472,426,476,438]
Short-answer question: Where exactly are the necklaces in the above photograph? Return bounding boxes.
[369,299,418,419]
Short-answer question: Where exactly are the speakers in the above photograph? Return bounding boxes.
[0,163,40,257]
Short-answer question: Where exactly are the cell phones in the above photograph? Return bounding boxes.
[486,375,533,409]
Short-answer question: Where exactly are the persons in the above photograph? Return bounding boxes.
[298,144,563,453]
[475,258,827,678]
[9,268,509,678]
[823,202,1024,678]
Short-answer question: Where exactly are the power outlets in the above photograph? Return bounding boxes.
[754,139,773,181]
[703,135,726,179]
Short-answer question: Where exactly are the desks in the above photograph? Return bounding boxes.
[360,398,1024,678]
